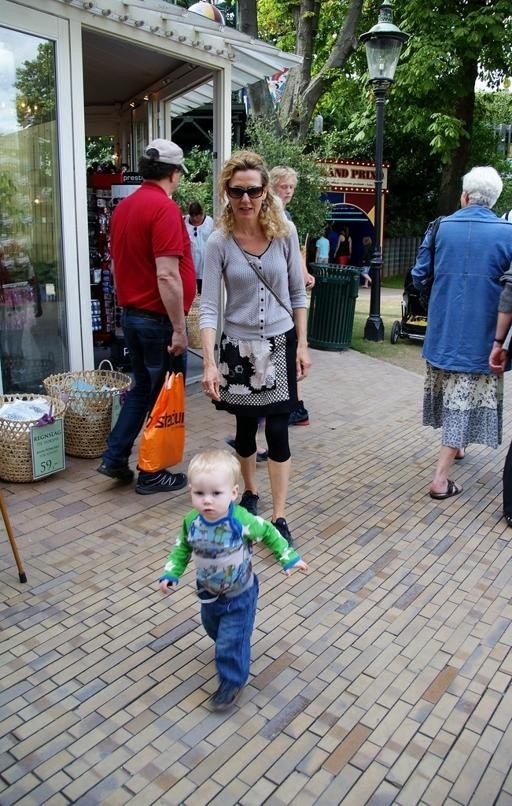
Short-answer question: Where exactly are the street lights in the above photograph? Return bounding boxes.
[358,0,409,342]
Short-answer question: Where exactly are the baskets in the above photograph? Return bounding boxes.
[43,358,132,460]
[0,385,66,482]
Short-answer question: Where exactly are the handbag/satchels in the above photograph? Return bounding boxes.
[419,276,434,311]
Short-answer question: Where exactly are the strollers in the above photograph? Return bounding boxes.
[392,267,433,343]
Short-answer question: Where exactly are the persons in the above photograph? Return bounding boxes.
[488,260,512,528]
[334,227,353,264]
[412,165,512,500]
[315,230,330,264]
[197,152,312,546]
[182,198,214,295]
[268,165,316,289]
[360,236,373,288]
[160,452,309,711]
[97,138,197,494]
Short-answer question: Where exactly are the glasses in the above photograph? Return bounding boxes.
[194,227,197,236]
[227,186,264,199]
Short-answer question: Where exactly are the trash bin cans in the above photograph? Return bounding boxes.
[306,262,366,351]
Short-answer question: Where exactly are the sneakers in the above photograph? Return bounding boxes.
[97,462,135,482]
[272,518,294,547]
[210,680,240,710]
[132,468,188,495]
[238,490,258,516]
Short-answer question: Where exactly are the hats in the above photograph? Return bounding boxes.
[143,139,189,174]
[189,203,202,213]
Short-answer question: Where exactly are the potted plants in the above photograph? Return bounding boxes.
[87,134,123,189]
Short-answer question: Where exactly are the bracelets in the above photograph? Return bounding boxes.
[494,339,504,343]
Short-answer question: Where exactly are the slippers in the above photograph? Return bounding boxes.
[430,478,463,499]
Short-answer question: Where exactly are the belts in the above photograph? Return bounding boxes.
[127,308,169,321]
